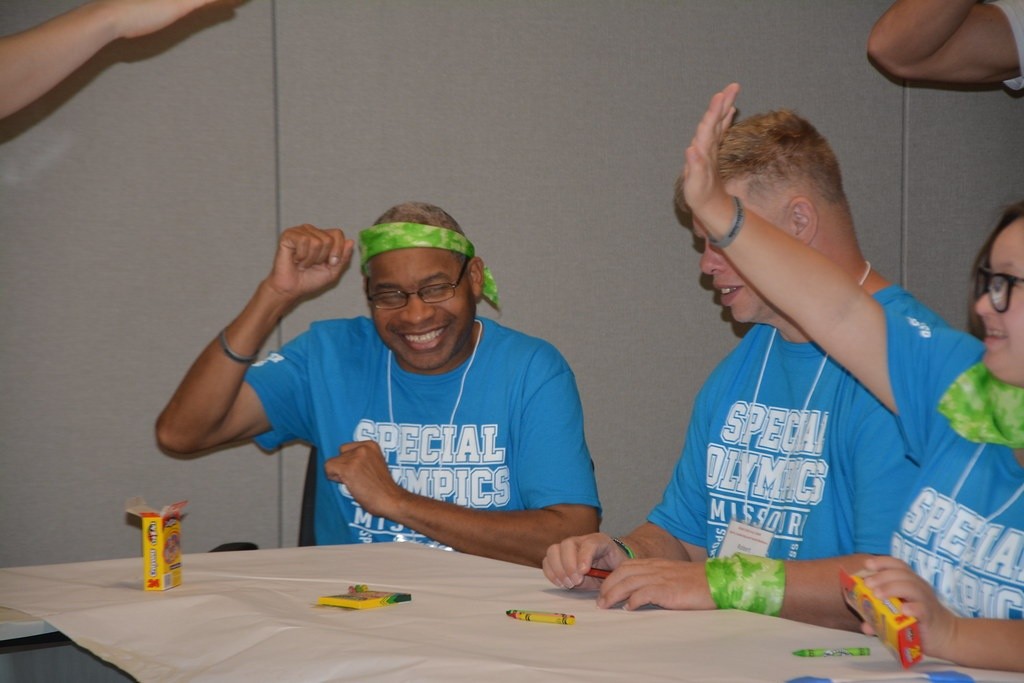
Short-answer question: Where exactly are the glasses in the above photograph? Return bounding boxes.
[974,266,1024,314]
[365,255,471,310]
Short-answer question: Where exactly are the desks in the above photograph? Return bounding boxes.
[1,542,1022,682]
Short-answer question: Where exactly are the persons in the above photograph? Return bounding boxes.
[867,0,1024,97]
[153,200,606,573]
[538,105,976,636]
[685,80,1023,675]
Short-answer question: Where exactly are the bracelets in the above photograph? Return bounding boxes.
[709,197,745,249]
[612,537,635,561]
[216,329,261,365]
